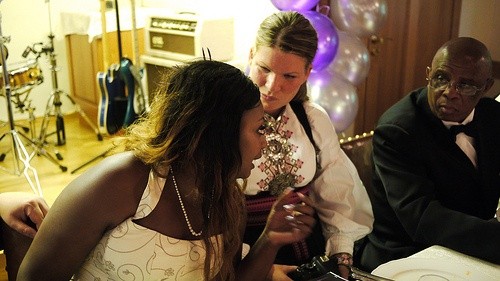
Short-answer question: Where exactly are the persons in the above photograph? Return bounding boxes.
[16,61,313,281]
[235,8,374,281]
[363,38,500,273]
[0,192,50,281]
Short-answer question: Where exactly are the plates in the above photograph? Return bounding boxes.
[370,258,481,281]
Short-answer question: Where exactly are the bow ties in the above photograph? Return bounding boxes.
[447,119,478,142]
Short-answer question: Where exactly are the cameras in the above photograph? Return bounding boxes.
[292,255,339,281]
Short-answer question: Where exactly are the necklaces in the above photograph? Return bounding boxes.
[169,168,210,236]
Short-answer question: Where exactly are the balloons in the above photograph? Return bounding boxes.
[270,0,321,11]
[297,11,339,71]
[312,75,359,131]
[329,0,388,39]
[306,81,312,99]
[325,29,371,85]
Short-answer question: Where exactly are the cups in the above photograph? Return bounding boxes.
[417,274,450,281]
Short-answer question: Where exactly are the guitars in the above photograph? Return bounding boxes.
[120,0,151,129]
[97,0,127,137]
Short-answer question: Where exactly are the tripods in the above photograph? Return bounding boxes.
[0,1,103,202]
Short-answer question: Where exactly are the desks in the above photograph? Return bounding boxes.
[62,10,147,125]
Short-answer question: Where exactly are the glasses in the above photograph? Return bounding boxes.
[426,68,489,96]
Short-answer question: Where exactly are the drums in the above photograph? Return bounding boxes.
[0,58,43,94]
